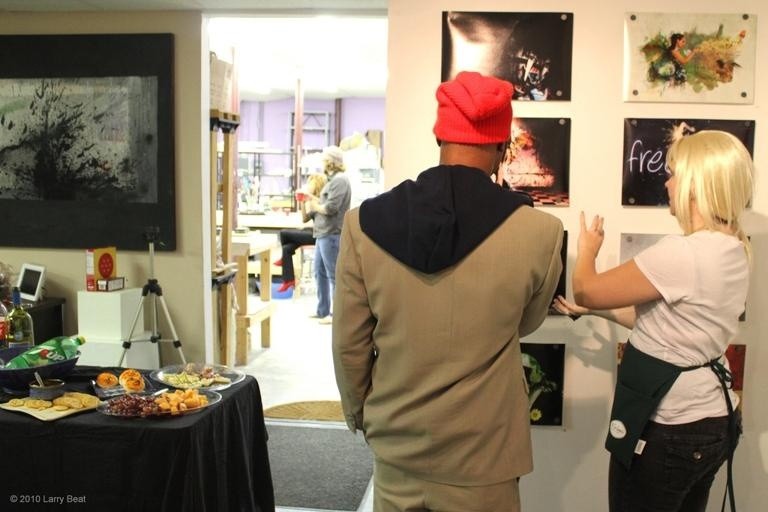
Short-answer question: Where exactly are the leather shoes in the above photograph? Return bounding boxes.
[308,311,319,317]
[319,314,332,324]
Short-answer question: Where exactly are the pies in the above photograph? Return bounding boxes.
[96,368,146,393]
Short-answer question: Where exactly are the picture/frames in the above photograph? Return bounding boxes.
[2,30,176,252]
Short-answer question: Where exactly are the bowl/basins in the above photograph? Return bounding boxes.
[0,347,82,397]
[27,377,65,401]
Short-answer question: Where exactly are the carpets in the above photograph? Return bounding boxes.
[262,400,346,421]
[256,423,375,512]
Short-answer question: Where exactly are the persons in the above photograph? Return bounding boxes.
[301,144,349,322]
[668,33,698,86]
[334,71,564,512]
[548,129,752,510]
[273,173,324,291]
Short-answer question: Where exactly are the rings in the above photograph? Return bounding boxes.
[596,228,605,235]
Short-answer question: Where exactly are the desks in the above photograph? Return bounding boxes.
[0,295,66,349]
[215,232,276,367]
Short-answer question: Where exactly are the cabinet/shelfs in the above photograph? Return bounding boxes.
[209,108,240,364]
[216,141,323,211]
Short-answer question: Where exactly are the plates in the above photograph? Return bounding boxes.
[0,390,104,423]
[95,387,221,420]
[90,372,151,397]
[149,362,247,391]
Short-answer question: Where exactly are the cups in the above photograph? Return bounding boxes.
[295,192,304,201]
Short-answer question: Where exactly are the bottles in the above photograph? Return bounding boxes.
[4,335,87,369]
[0,297,12,340]
[7,286,36,345]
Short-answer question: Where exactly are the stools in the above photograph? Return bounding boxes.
[299,246,318,293]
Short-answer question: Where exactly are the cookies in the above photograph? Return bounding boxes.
[8,391,99,413]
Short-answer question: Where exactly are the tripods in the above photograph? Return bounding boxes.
[118,243,187,368]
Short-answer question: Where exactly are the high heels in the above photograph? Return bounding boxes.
[277,279,294,292]
[274,260,282,265]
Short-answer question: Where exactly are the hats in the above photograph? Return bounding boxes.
[433,70,514,146]
[323,145,344,166]
